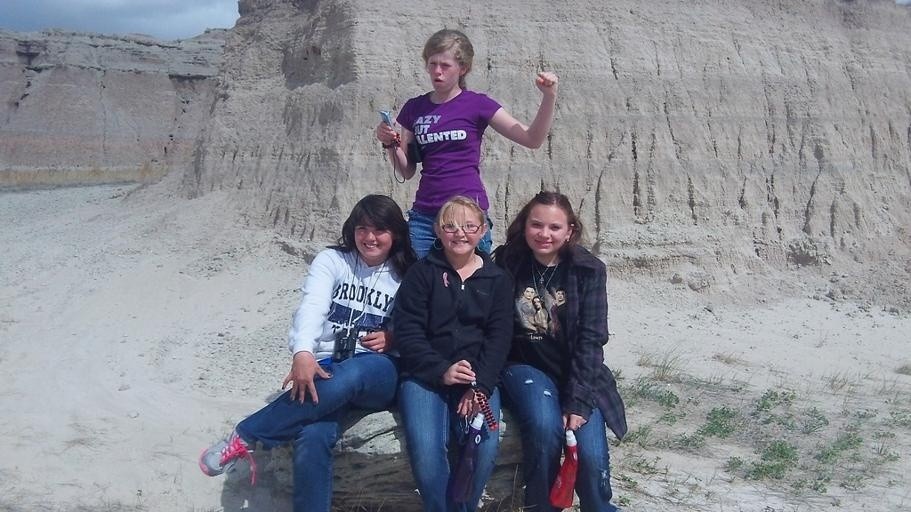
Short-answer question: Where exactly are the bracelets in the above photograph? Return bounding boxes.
[382,143,393,149]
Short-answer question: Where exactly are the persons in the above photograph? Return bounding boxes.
[199,195,417,510]
[516,285,538,332]
[491,191,628,512]
[376,29,559,262]
[551,287,568,336]
[394,193,515,511]
[528,294,549,334]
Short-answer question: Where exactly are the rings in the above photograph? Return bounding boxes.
[576,424,581,428]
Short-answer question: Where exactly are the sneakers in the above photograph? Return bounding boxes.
[199,430,254,476]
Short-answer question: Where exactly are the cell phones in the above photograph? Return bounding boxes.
[379,109,397,136]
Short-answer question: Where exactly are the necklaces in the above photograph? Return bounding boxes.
[534,254,558,284]
[531,252,561,303]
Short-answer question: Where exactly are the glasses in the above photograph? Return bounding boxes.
[440,223,482,234]
